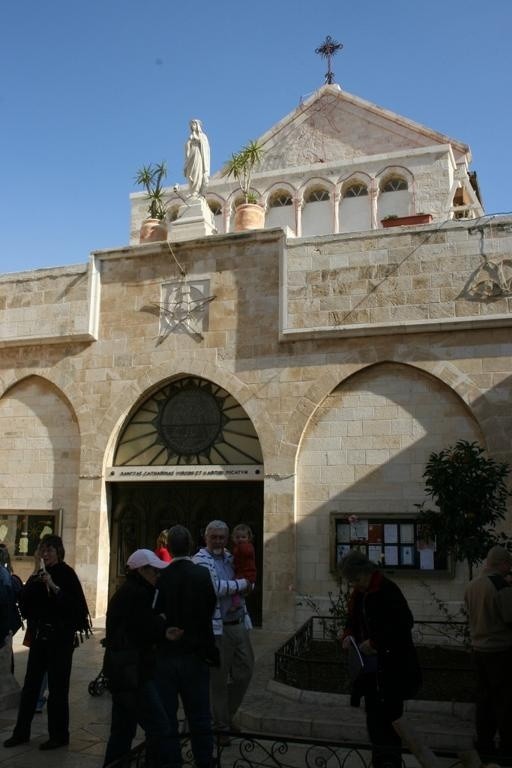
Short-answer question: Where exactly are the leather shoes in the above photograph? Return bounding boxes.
[216,730,230,746]
[4,734,30,746]
[39,738,65,750]
[232,727,244,739]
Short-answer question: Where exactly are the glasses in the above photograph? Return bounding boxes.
[40,549,57,554]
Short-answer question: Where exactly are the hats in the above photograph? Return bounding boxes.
[481,547,511,576]
[127,550,169,571]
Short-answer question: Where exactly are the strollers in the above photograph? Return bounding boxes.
[88,639,108,696]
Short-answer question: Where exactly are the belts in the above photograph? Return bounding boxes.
[223,618,245,627]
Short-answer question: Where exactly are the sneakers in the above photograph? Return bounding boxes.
[35,700,43,713]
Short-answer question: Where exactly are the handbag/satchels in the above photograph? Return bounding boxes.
[330,635,378,696]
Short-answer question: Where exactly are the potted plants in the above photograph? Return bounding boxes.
[133,158,172,242]
[224,138,266,233]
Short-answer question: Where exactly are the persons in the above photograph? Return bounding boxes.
[232,524,260,622]
[332,551,414,768]
[4,533,89,751]
[153,528,218,768]
[150,526,190,562]
[463,547,512,768]
[102,549,173,766]
[193,520,255,732]
[0,543,23,680]
